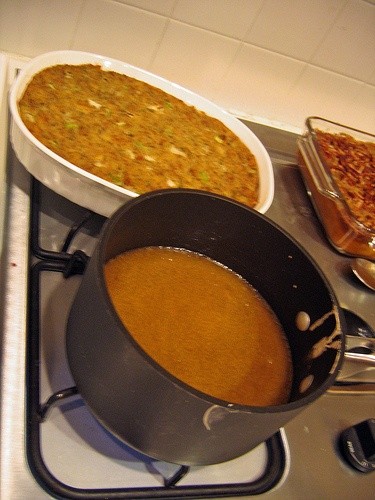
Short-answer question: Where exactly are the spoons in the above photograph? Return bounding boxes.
[340,305,375,340]
[350,258,375,291]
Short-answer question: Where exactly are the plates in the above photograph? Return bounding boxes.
[302,114,375,256]
[8,51,275,217]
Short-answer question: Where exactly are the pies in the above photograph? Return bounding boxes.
[19,63,260,210]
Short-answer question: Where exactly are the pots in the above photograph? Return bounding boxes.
[65,189,347,469]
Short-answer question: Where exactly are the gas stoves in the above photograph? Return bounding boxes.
[1,53,375,500]
